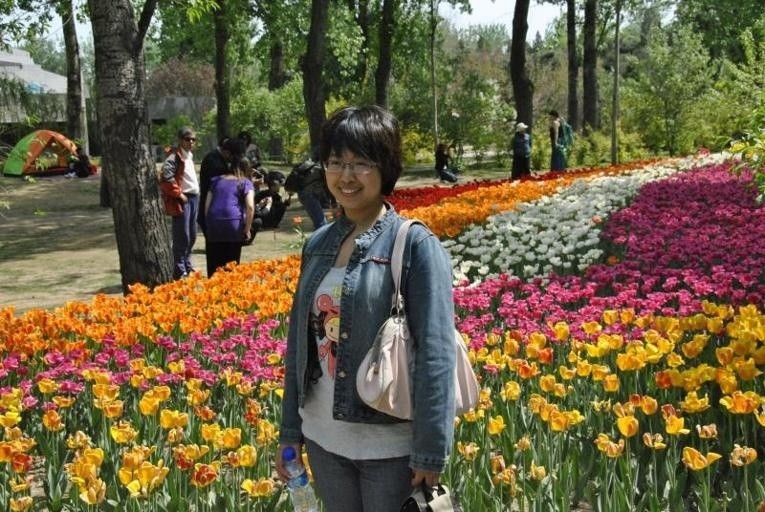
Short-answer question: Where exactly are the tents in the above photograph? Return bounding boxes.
[2,129,85,178]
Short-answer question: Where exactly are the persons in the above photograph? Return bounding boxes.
[435,144,457,183]
[197,131,291,281]
[274,104,455,510]
[548,110,572,171]
[64,148,93,179]
[159,125,201,282]
[295,163,338,232]
[510,122,532,178]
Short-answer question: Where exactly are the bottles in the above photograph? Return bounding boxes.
[280,446,319,511]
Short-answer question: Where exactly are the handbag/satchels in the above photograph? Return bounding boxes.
[355,314,480,421]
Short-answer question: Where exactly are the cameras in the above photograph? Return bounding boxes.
[450,144,456,148]
[253,169,266,179]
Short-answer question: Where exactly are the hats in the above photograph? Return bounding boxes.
[516,122,529,132]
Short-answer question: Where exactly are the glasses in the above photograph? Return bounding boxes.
[324,155,379,175]
